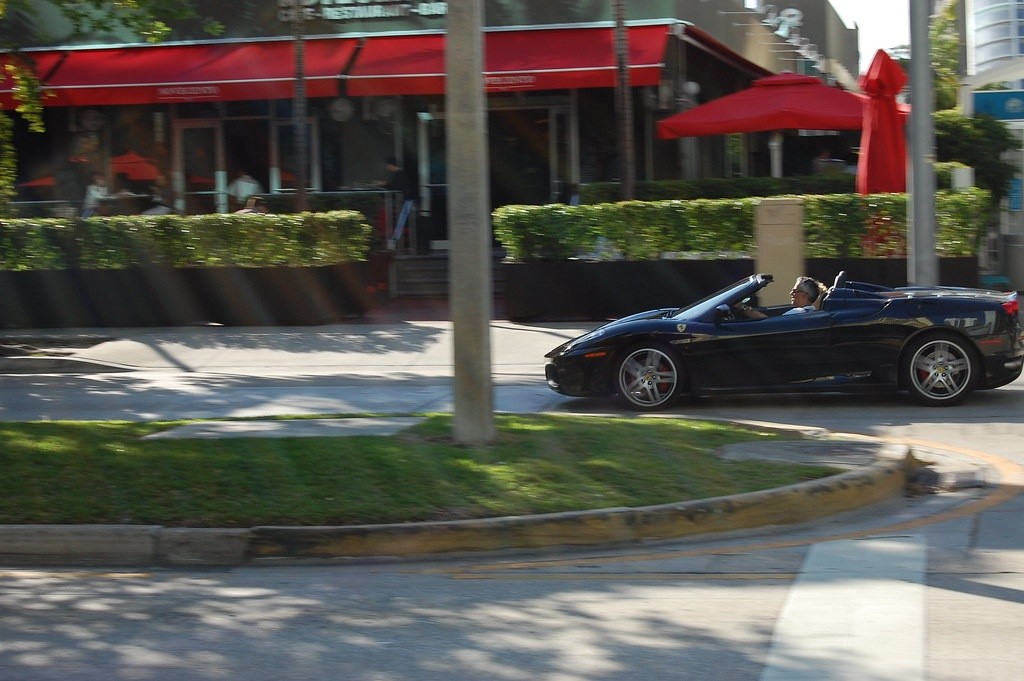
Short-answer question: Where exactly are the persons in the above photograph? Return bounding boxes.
[79,172,178,216]
[234,195,267,216]
[226,168,263,213]
[373,155,418,200]
[734,276,820,320]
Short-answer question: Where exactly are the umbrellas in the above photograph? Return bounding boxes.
[655,70,911,180]
[855,46,908,256]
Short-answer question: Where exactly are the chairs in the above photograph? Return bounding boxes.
[819,271,846,308]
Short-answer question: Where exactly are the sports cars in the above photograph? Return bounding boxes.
[544,271,1024,415]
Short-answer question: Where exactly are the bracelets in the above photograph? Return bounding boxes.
[737,302,752,312]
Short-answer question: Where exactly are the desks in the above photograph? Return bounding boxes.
[8,201,69,203]
[340,186,388,190]
[99,193,152,214]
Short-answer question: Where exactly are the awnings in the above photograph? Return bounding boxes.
[0,26,670,110]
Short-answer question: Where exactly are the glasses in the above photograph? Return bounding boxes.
[789,288,799,293]
